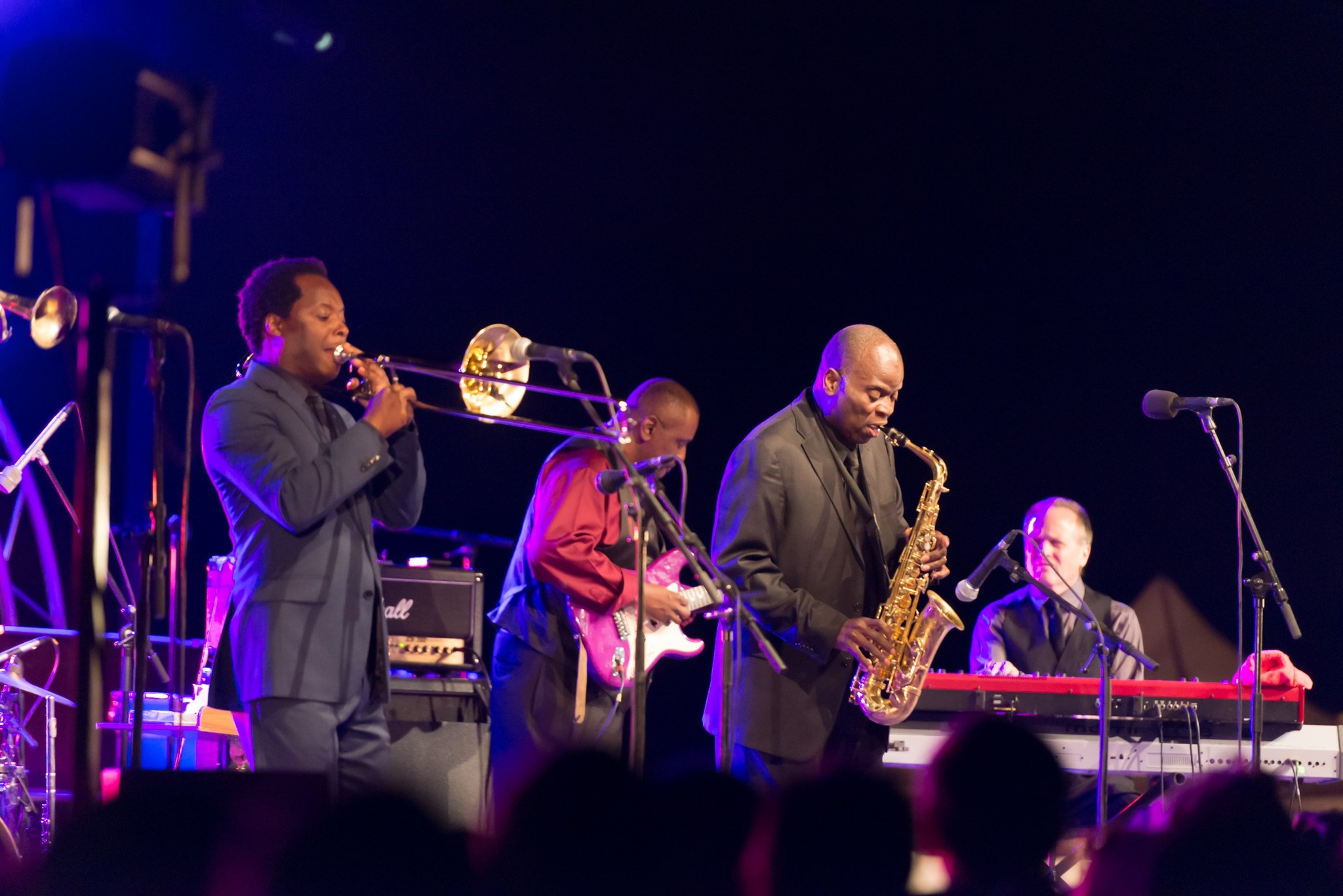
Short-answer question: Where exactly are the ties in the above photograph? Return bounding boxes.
[846,451,865,554]
[307,389,331,442]
[1043,598,1065,660]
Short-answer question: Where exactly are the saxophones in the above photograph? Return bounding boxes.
[850,424,964,726]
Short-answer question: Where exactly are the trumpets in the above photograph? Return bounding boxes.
[0,285,78,350]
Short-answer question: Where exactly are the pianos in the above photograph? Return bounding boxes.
[875,664,1343,896]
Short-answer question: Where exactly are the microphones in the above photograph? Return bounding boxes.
[956,529,1018,602]
[1142,390,1235,420]
[0,635,51,662]
[106,306,184,335]
[594,454,674,494]
[0,402,75,494]
[509,337,592,362]
[14,187,39,277]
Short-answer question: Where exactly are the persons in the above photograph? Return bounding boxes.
[969,496,1144,718]
[487,372,701,828]
[200,255,427,801]
[38,722,1343,896]
[700,323,950,777]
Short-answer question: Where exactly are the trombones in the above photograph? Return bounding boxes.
[235,323,631,443]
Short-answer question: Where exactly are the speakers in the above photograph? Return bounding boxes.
[196,677,491,836]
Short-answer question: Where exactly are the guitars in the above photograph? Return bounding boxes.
[565,546,727,688]
